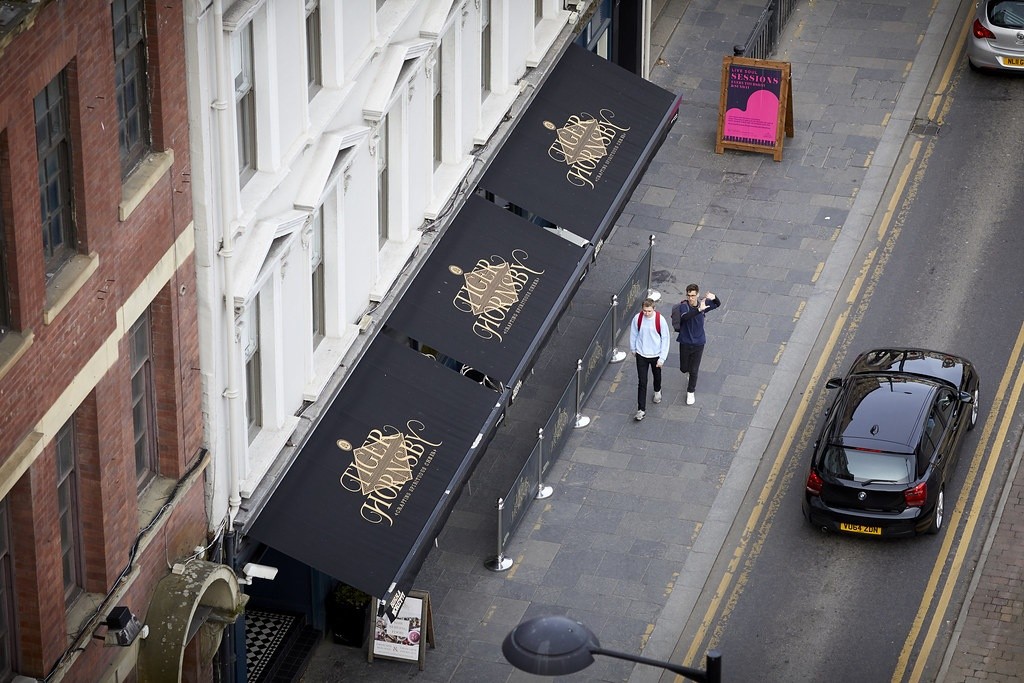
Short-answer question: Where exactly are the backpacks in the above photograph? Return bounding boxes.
[671,299,701,332]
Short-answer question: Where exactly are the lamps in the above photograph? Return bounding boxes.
[92,606,141,646]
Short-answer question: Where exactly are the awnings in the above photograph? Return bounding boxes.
[387,193,595,409]
[247,330,512,623]
[477,42,683,262]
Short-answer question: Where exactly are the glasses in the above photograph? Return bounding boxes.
[687,295,697,297]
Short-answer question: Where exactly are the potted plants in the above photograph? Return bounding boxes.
[326,582,371,648]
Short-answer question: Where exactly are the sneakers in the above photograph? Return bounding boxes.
[687,392,695,405]
[682,372,689,380]
[634,409,645,421]
[652,390,662,404]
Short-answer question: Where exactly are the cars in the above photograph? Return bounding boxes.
[967,0,1024,75]
[800,347,980,539]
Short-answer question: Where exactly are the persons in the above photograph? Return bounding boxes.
[671,285,721,405]
[628,299,670,421]
